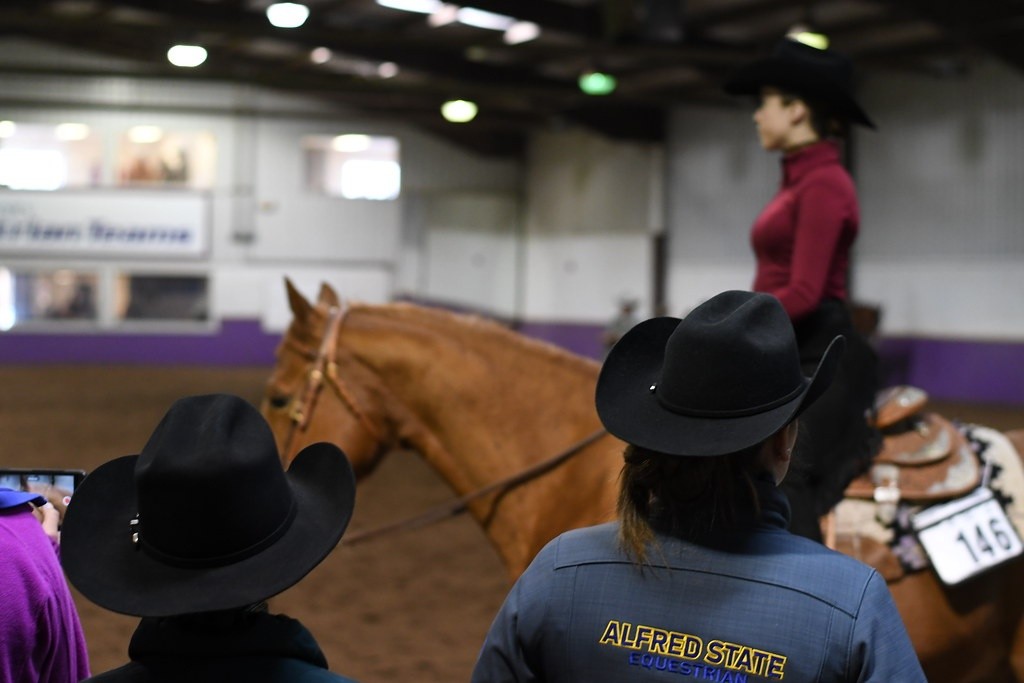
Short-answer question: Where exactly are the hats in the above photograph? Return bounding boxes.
[61,393,356,620]
[692,38,877,131]
[595,291,849,457]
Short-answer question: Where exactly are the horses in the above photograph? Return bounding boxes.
[260,277,1024,682]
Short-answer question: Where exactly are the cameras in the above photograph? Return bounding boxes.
[0,468,87,547]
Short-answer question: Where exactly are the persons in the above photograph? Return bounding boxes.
[0,488,89,683]
[748,32,878,548]
[468,290,930,683]
[57,394,357,683]
